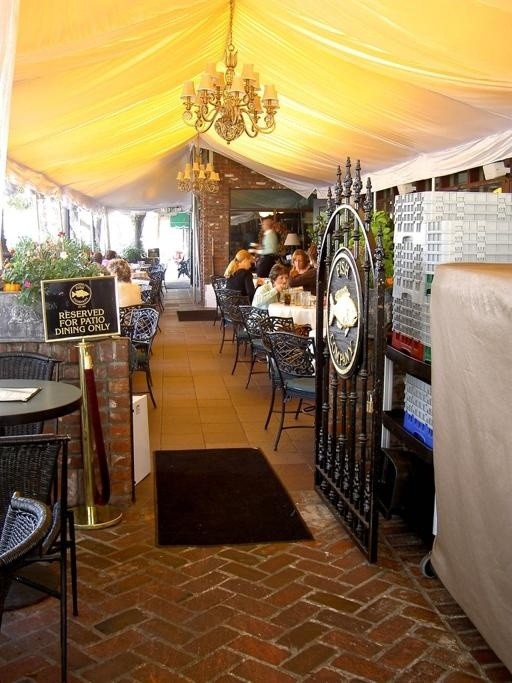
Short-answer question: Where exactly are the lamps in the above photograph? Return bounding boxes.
[176,133,220,198]
[284,233,301,256]
[180,0,280,146]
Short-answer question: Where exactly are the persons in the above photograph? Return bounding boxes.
[101,250,117,270]
[242,264,291,332]
[221,249,261,323]
[243,245,259,280]
[103,260,143,308]
[289,244,318,301]
[289,249,314,277]
[276,243,292,267]
[90,254,110,276]
[248,218,279,278]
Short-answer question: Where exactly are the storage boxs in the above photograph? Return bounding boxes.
[402,371,433,430]
[392,192,512,363]
[403,411,432,448]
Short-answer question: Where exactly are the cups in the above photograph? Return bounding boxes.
[280,288,300,306]
[300,291,311,308]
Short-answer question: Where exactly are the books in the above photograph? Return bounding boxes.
[0,387,42,403]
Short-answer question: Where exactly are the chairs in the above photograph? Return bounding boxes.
[0,351,65,562]
[149,278,165,312]
[121,324,135,337]
[140,290,161,333]
[119,304,156,355]
[0,433,78,683]
[257,316,315,430]
[266,331,316,451]
[214,289,248,354]
[210,275,225,326]
[0,490,52,625]
[158,269,167,293]
[211,276,238,331]
[224,296,261,376]
[129,307,160,408]
[238,305,271,389]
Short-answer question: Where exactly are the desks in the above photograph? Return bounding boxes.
[1,380,83,610]
[266,303,315,377]
